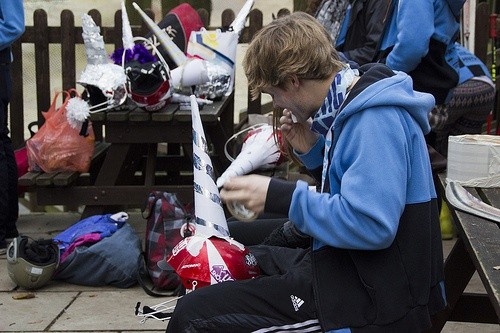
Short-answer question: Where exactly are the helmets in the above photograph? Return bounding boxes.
[7,235,60,291]
[76,62,128,114]
[124,37,173,114]
[157,235,259,295]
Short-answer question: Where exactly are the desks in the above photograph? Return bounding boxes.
[80,88,235,220]
[435,169,500,321]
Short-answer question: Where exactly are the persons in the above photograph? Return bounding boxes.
[0,0,25,256]
[337,0,496,239]
[164,10,445,332]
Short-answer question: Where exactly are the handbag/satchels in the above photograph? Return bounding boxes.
[26,88,95,173]
[58,213,143,288]
[187,26,238,97]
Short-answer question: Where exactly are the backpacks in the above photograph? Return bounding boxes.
[138,192,195,297]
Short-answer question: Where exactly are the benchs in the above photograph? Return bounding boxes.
[18,137,110,189]
[251,157,291,179]
